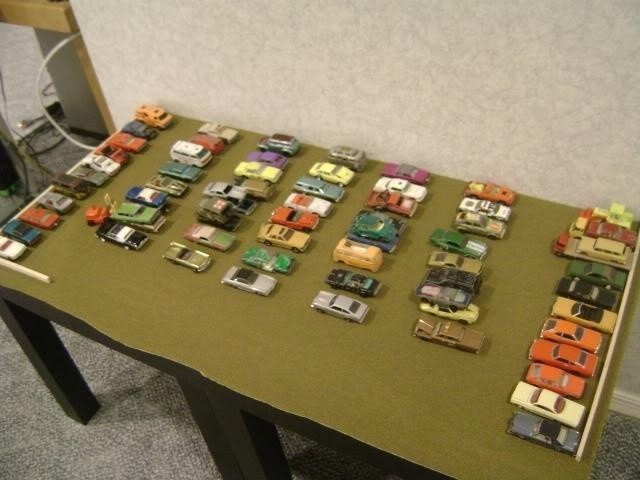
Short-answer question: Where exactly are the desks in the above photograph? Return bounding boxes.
[0,109,640,479]
